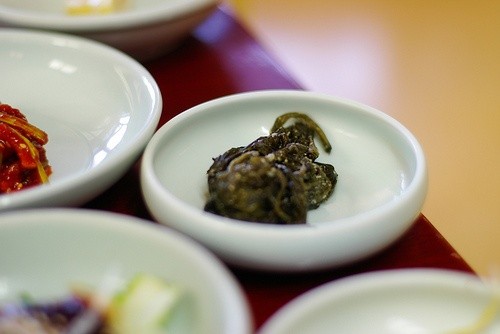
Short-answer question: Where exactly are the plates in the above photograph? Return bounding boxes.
[258,268,500,334]
[0,0,222,61]
[141,88,428,272]
[0,26,163,213]
[1,208,252,334]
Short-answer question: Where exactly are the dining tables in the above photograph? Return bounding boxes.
[71,9,477,334]
